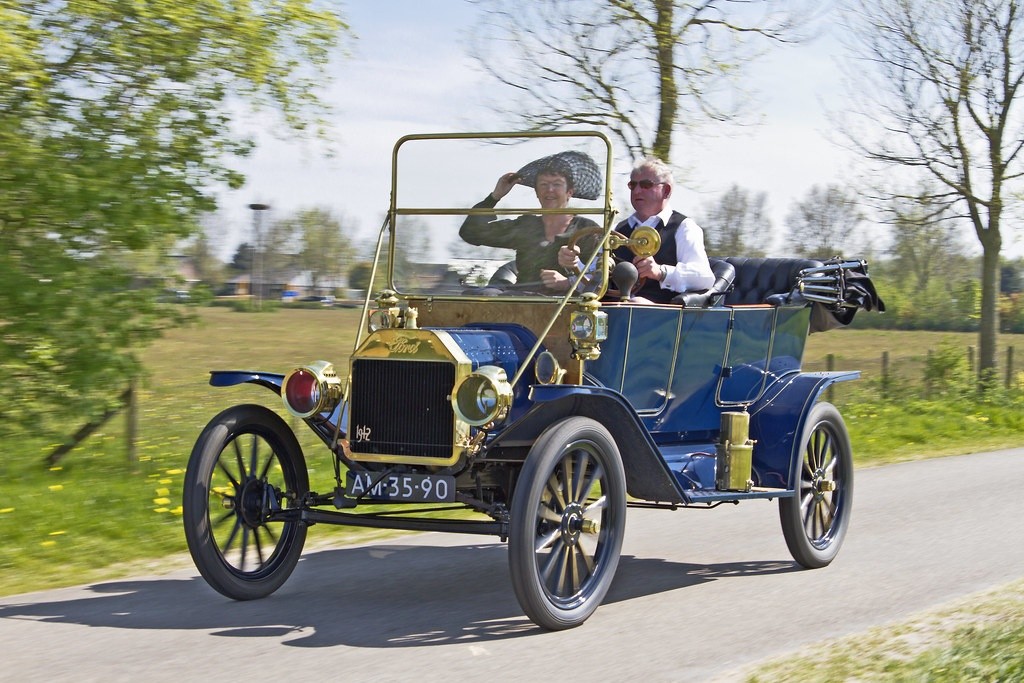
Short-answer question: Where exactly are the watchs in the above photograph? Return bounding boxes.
[659,264,667,282]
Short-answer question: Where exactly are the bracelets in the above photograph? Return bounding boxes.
[491,194,500,200]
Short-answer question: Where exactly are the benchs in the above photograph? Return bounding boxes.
[489,256,825,306]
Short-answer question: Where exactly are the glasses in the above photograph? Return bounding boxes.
[627,179,666,191]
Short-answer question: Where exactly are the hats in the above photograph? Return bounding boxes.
[509,149,602,201]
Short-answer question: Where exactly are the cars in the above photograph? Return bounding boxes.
[182,130,888,630]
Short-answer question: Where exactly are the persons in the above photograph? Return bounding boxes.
[558,155,716,304]
[459,151,602,296]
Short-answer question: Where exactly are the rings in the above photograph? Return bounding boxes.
[569,250,573,256]
